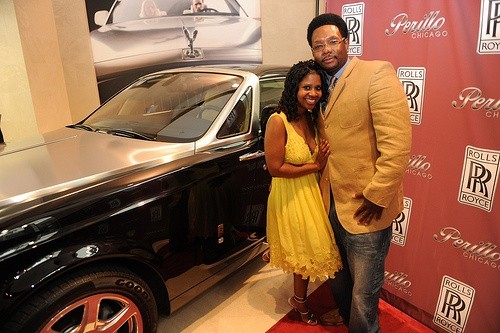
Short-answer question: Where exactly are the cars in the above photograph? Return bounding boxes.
[0,64,297,333]
[89,0,262,93]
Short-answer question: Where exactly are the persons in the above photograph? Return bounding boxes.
[307,12,413,333]
[262,59,330,326]
[191,0,206,15]
[139,0,160,18]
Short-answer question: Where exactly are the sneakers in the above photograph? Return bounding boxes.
[322,307,346,326]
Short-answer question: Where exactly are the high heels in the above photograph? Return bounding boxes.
[288,294,318,326]
[262,240,272,263]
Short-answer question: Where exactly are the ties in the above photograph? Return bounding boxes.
[325,76,337,113]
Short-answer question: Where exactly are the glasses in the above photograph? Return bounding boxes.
[309,35,346,54]
